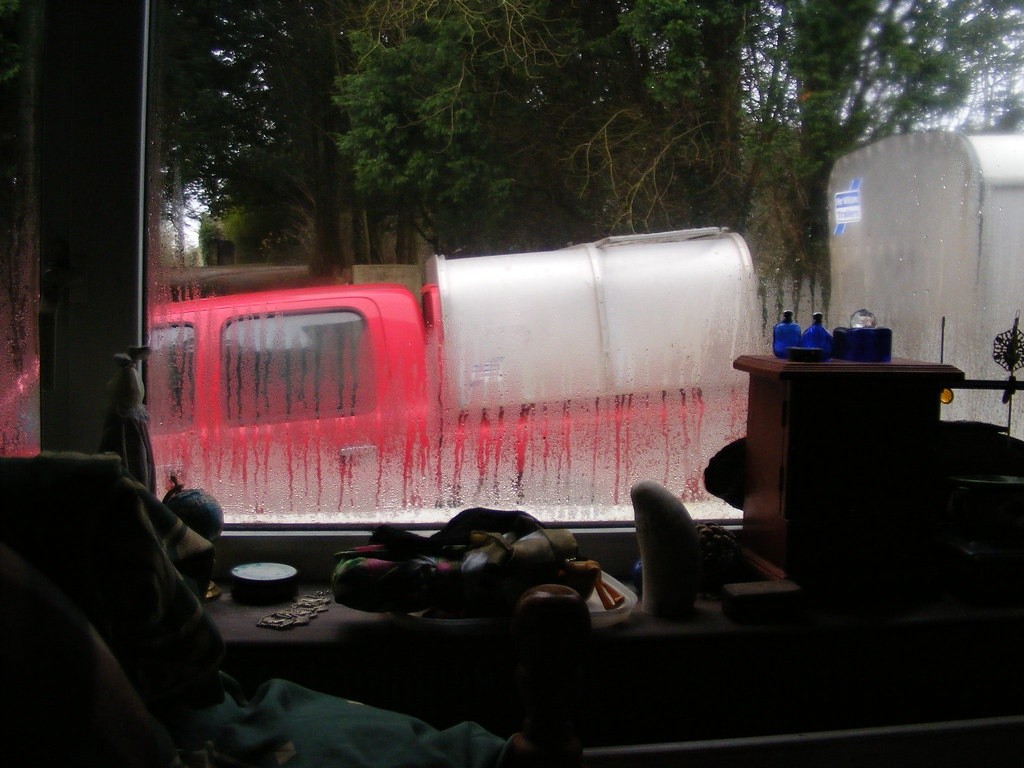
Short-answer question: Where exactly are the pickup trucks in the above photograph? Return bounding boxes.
[1,276,746,518]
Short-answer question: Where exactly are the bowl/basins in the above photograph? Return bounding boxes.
[787,347,822,361]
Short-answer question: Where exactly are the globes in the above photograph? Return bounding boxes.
[159,475,225,602]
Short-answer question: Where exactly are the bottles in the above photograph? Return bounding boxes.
[798,313,833,361]
[773,310,800,358]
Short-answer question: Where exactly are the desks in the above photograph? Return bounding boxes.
[734,355,963,595]
